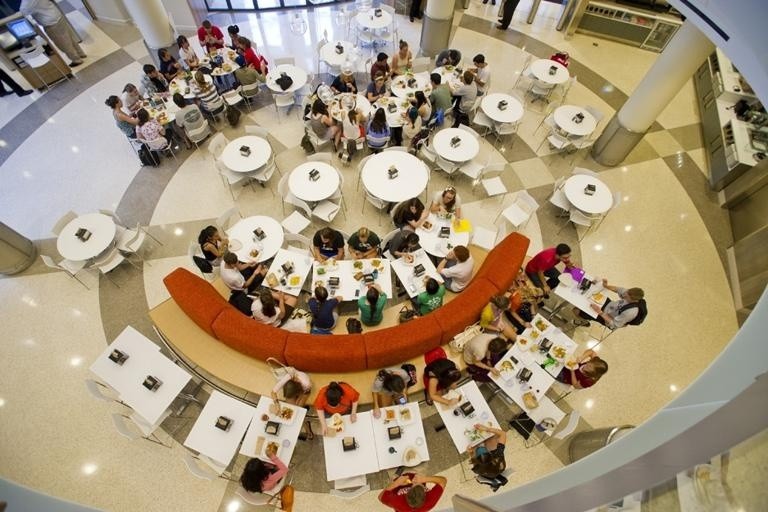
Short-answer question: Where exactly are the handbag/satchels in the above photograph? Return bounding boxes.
[138,143,160,168]
[266,357,294,381]
[223,96,241,126]
[400,363,418,387]
[398,305,416,322]
[448,321,483,354]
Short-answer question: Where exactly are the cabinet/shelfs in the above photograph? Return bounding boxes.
[576,0,685,55]
[692,56,729,192]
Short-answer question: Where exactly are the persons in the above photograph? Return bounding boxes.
[103,19,269,158]
[376,465,447,512]
[19,1,87,68]
[466,423,508,479]
[573,277,645,331]
[555,349,608,390]
[409,0,422,22]
[238,443,289,494]
[196,186,574,436]
[0,69,33,97]
[301,40,491,153]
[495,0,520,30]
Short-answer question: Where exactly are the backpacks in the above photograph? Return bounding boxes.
[618,299,648,326]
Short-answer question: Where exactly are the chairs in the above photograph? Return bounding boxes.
[18,43,72,93]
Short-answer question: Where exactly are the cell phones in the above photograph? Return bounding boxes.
[511,357,518,364]
[355,290,359,296]
[399,395,407,405]
[330,290,335,296]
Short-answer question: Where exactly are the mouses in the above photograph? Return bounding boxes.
[25,43,32,48]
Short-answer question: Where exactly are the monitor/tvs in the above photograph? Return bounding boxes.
[7,18,37,40]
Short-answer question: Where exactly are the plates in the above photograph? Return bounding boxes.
[262,440,281,460]
[420,220,434,232]
[118,47,239,123]
[260,403,412,431]
[557,271,607,306]
[495,320,579,409]
[320,65,463,118]
[228,238,426,287]
[467,421,496,437]
[403,448,421,466]
